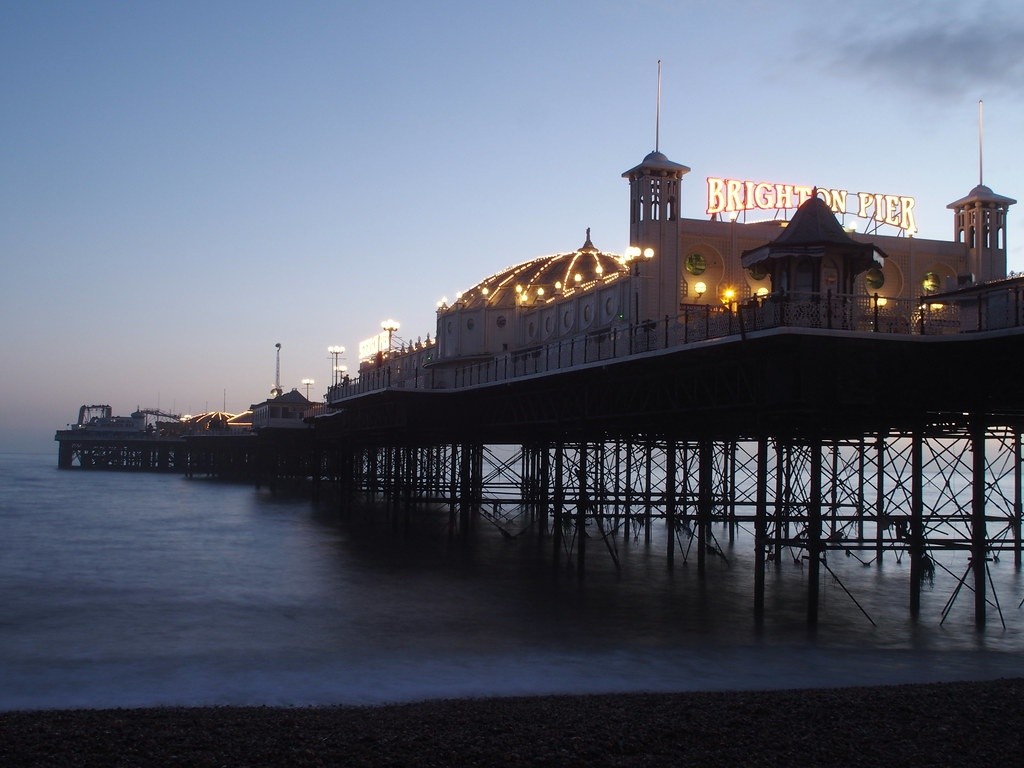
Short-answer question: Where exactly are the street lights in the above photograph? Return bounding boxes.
[329,345,345,386]
[301,379,315,402]
[334,367,346,382]
[381,320,400,353]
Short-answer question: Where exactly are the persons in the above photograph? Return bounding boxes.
[341,374,349,388]
